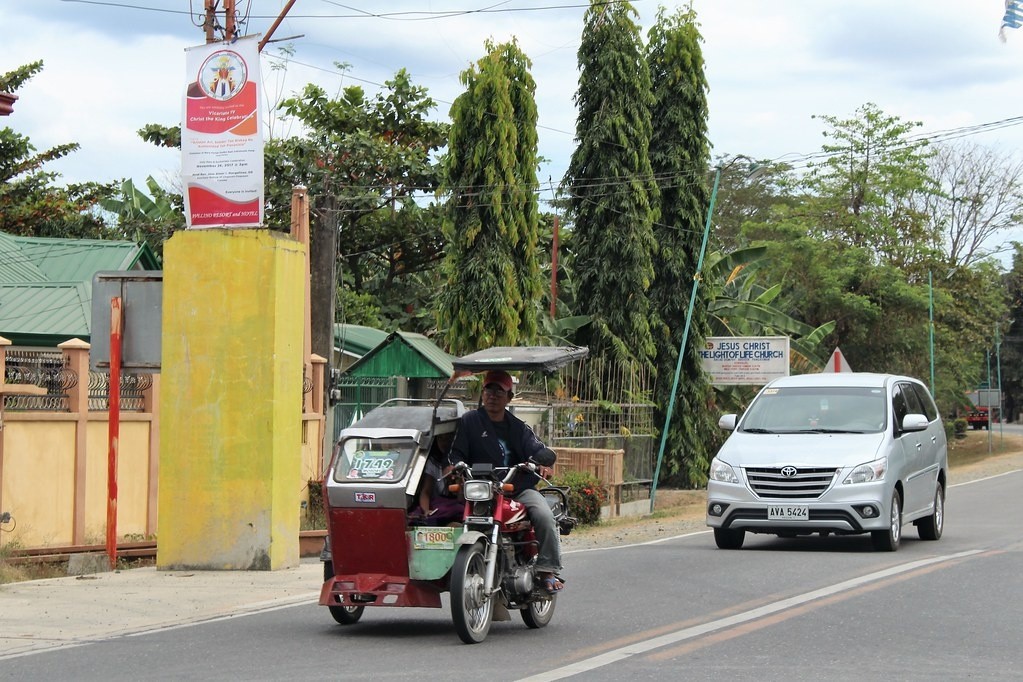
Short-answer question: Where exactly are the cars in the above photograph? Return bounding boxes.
[705,370,948,552]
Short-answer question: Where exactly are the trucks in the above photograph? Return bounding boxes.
[965,389,993,430]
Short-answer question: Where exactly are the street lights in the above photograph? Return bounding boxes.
[928,265,958,402]
[994,319,1015,452]
[648,152,770,514]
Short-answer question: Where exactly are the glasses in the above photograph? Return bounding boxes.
[483,387,508,397]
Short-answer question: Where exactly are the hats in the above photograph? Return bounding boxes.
[484,369,513,392]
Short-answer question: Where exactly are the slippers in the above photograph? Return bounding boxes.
[541,573,562,594]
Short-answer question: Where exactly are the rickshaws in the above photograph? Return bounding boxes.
[315,345,589,645]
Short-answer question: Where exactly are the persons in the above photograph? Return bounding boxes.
[448,370,566,594]
[372,431,466,526]
